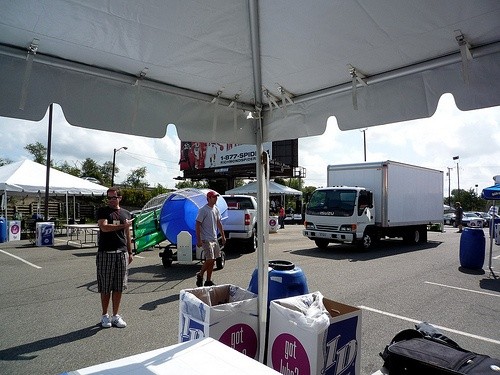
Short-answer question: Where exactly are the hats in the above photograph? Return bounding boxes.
[207,192,219,198]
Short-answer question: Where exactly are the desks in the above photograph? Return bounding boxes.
[63,336,282,374]
[63,223,99,248]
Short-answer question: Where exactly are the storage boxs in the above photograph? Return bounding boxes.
[266,291,363,375]
[179,284,259,360]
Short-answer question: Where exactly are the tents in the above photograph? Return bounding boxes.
[481,175,500,269]
[225,180,302,220]
[0,0,500,363]
[0,158,110,239]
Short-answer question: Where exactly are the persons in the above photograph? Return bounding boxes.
[288,206,294,214]
[279,206,285,229]
[454,202,463,233]
[96,187,134,328]
[196,191,226,288]
[270,198,280,214]
[296,198,301,214]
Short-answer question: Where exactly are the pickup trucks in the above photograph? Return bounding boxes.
[219,193,258,250]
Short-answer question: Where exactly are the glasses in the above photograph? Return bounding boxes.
[107,196,118,200]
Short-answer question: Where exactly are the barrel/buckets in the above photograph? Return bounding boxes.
[459,226,486,268]
[247,259,310,359]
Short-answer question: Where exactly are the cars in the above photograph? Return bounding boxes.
[443,204,499,227]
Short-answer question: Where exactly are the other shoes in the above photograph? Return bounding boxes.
[457,230,462,233]
[204,281,216,286]
[196,273,203,287]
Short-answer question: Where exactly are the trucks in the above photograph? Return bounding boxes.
[302,160,446,250]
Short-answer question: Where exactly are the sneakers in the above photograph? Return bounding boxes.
[102,314,112,327]
[111,315,127,328]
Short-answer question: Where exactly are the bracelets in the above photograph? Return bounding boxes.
[128,252,133,254]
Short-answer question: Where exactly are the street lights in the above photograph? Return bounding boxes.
[111,145,128,187]
[475,184,478,196]
[447,167,452,206]
[453,156,460,199]
